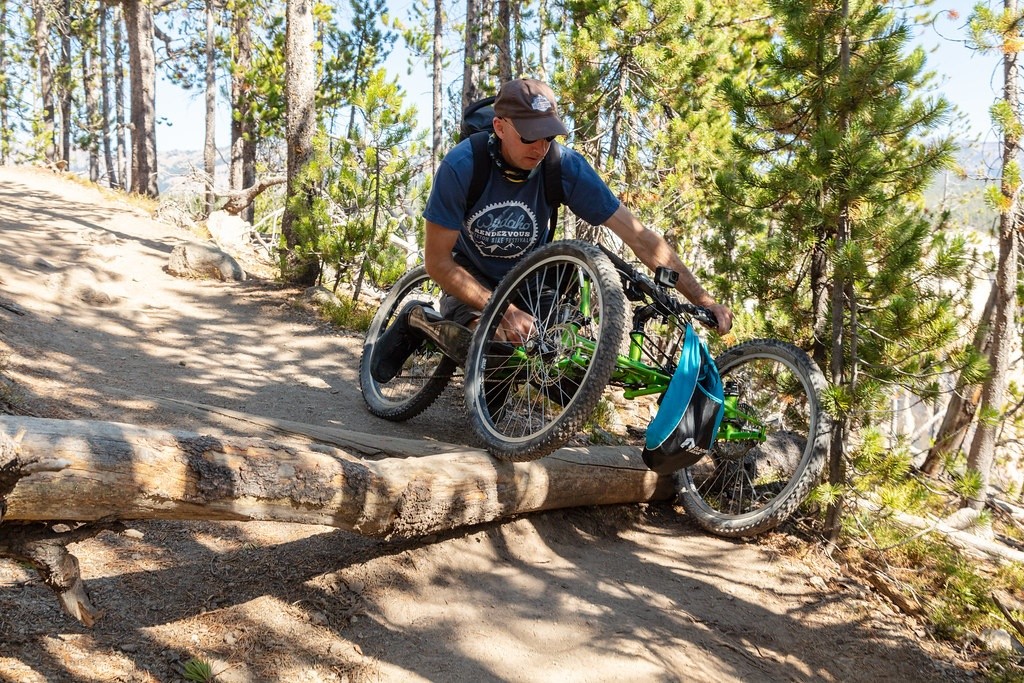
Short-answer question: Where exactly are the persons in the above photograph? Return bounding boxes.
[369,77,733,384]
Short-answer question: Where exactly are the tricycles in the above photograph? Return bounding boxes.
[360,241,832,537]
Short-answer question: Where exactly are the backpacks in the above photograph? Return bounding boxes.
[459,96,562,243]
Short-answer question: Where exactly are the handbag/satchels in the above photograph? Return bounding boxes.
[641,307,725,473]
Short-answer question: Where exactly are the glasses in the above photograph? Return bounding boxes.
[502,118,556,145]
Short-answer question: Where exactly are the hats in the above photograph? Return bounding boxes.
[494,77,570,141]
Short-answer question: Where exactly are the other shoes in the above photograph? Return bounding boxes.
[484,377,518,422]
[370,300,435,383]
